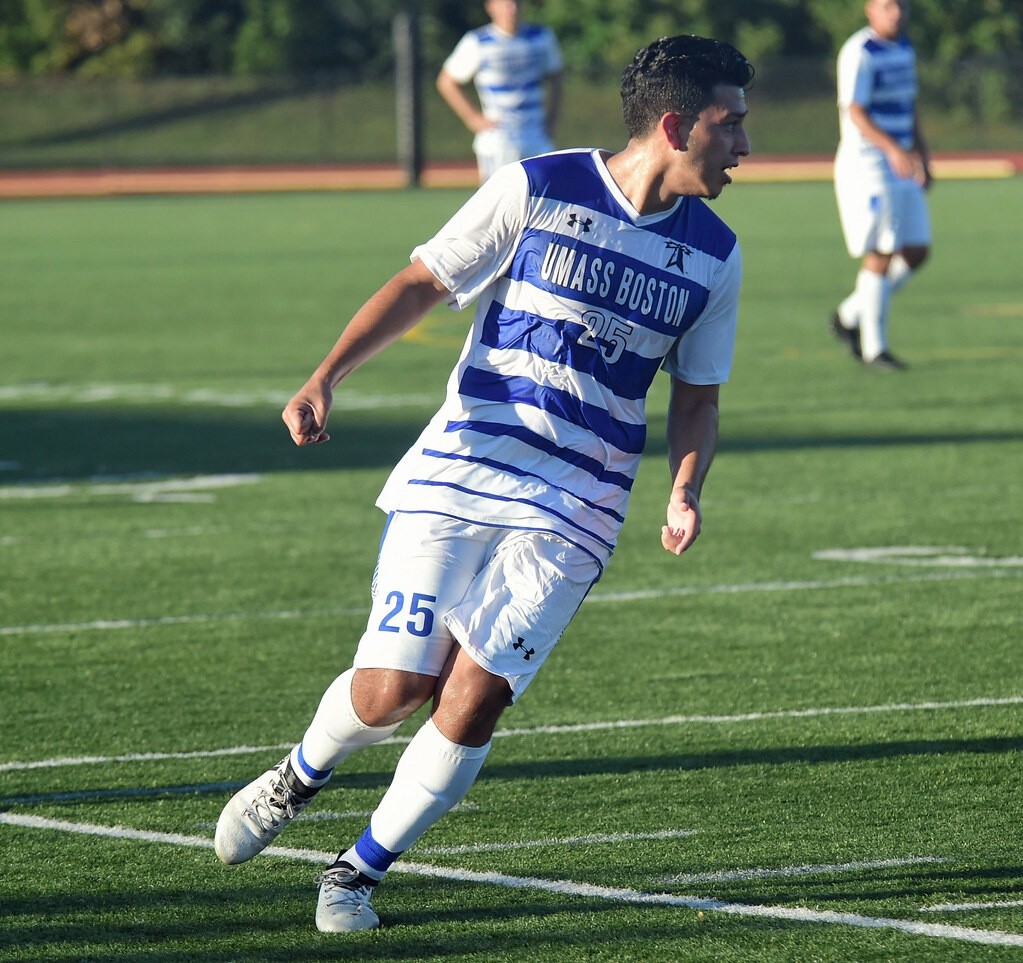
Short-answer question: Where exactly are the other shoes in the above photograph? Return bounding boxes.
[831,304,863,359]
[867,352,907,377]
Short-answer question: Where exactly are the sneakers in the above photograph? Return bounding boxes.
[314,849,380,934]
[213,747,321,865]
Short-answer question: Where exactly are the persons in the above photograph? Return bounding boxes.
[213,34,755,935]
[434,0,567,190]
[830,0,935,375]
